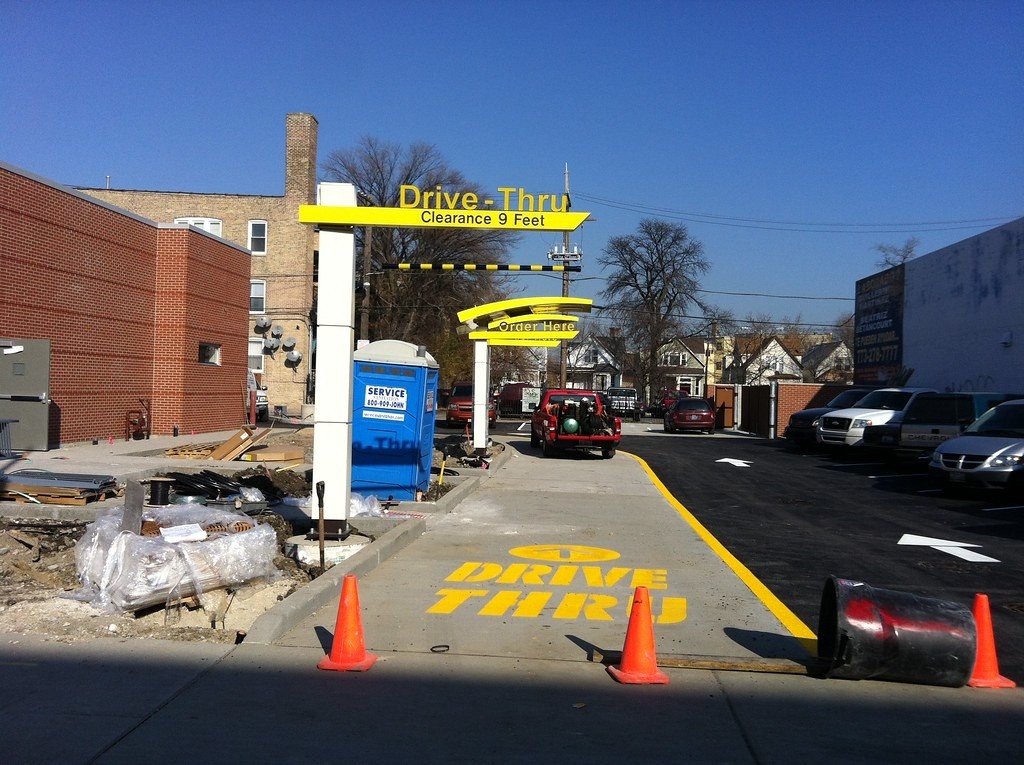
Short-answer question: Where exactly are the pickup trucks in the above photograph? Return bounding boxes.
[607,386,643,422]
[649,390,690,418]
[528,389,621,460]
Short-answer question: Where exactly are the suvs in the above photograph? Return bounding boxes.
[254,380,269,421]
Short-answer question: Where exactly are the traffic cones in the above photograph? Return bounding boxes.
[967,592,1017,690]
[606,585,670,687]
[317,573,380,671]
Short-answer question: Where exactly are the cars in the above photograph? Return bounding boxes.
[927,397,1024,499]
[815,388,938,451]
[663,397,717,435]
[444,380,498,425]
[862,392,1024,488]
[787,390,872,442]
